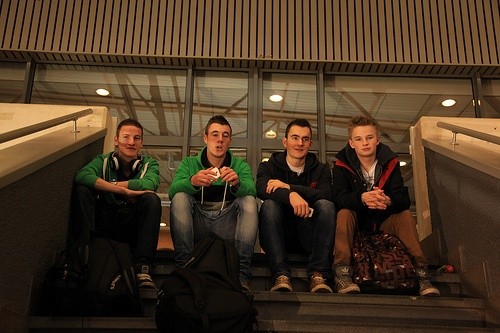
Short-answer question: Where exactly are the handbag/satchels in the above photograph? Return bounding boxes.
[32,251,81,315]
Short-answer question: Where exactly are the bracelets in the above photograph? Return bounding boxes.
[115,181,118,185]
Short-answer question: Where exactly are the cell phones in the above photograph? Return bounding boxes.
[207,167,221,182]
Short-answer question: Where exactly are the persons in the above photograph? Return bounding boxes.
[72,119,162,291]
[169,115,258,292]
[332,114,440,296]
[255,118,335,293]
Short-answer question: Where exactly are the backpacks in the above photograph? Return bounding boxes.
[352,233,420,294]
[154,241,258,333]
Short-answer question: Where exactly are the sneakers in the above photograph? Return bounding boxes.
[334,265,360,294]
[308,274,333,292]
[270,275,293,292]
[417,266,441,297]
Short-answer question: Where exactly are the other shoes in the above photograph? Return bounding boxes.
[135,272,155,287]
